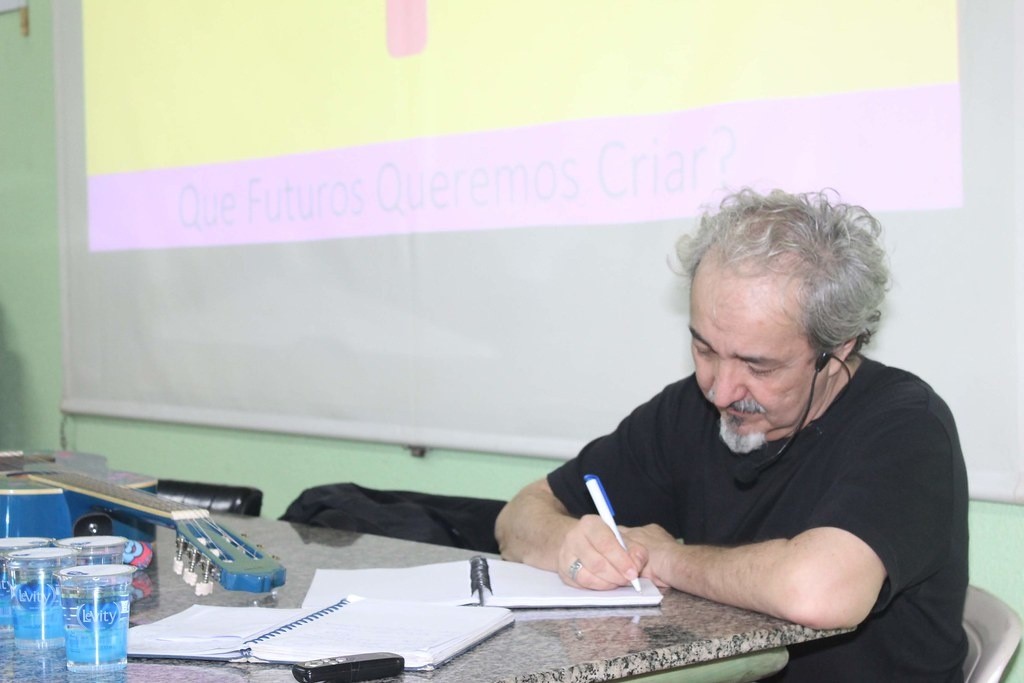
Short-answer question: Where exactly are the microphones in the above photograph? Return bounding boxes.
[735,352,831,486]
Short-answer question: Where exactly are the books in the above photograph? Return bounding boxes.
[301,557,664,610]
[127,591,515,671]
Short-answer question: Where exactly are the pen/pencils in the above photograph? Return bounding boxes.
[6,471,58,477]
[583,474,642,593]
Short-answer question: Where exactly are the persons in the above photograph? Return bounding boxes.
[490,186,970,683]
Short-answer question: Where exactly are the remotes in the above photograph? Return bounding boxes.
[292,651,405,683]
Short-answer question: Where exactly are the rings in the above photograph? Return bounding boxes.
[569,561,582,582]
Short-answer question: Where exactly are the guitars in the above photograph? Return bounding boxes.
[0,448,287,595]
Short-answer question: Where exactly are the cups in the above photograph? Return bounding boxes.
[4,546,79,649]
[0,537,51,632]
[53,535,129,565]
[53,562,137,672]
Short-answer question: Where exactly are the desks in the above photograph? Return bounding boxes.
[0,450,859,682]
